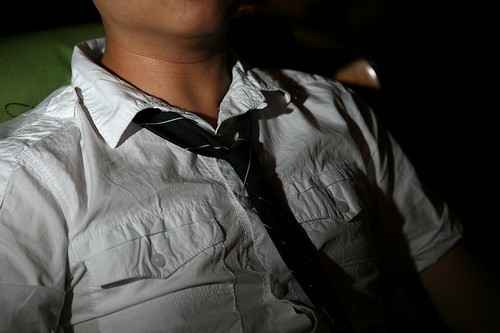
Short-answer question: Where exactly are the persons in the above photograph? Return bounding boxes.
[0,0,499,333]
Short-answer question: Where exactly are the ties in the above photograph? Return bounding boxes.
[134,108,344,333]
